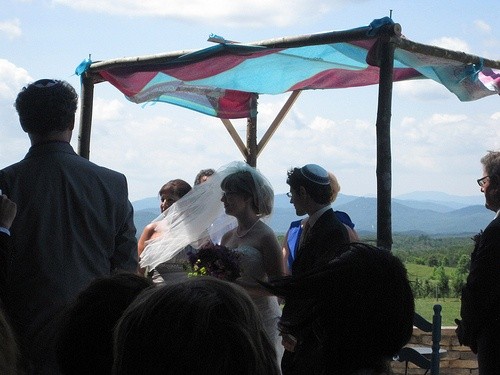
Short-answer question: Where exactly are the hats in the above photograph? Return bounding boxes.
[300,164,330,185]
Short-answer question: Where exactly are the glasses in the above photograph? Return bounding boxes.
[476,175,491,187]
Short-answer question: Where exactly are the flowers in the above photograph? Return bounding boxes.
[183,240,244,283]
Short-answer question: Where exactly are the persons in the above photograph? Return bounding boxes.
[454,151,500,375]
[0,78,415,375]
